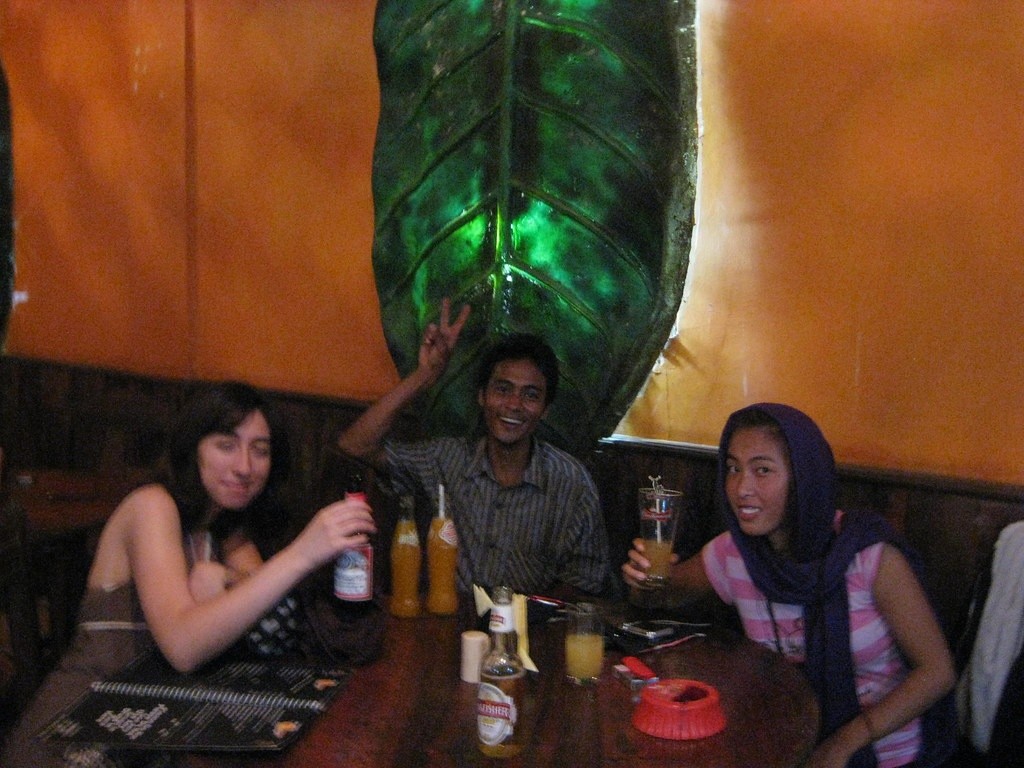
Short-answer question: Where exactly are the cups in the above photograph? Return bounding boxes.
[638,487,682,582]
[565,600,605,686]
[461,631,490,683]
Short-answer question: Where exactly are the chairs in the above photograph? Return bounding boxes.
[949,519,1024,768]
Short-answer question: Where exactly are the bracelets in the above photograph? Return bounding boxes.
[854,710,877,742]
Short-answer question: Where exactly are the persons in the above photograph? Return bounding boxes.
[336,295,611,621]
[0,379,377,768]
[621,402,957,768]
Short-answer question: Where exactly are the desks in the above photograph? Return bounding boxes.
[173,592,821,768]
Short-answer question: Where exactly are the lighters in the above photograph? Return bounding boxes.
[621,655,658,681]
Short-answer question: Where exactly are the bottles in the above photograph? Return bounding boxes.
[426,493,459,616]
[331,471,375,622]
[388,495,421,618]
[478,585,527,758]
